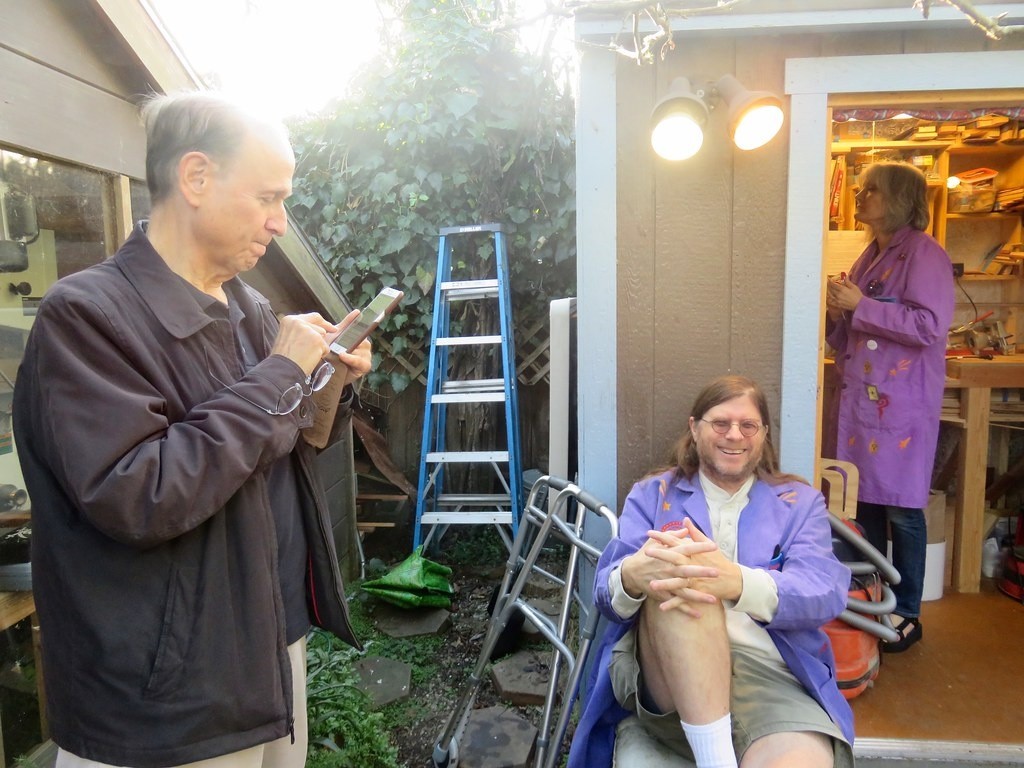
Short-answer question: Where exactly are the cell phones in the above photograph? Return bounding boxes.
[328,286,404,355]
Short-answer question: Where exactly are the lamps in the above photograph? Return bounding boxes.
[648,73,784,160]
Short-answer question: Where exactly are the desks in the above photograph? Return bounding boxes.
[945,353,1024,594]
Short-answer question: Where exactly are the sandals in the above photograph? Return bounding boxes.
[878,611,922,653]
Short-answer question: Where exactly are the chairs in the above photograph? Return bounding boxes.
[615,456,859,768]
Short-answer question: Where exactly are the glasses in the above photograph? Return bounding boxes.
[204,309,335,415]
[701,417,765,437]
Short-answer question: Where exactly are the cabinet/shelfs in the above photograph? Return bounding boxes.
[814,87,1024,491]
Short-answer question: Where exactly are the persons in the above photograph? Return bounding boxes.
[825,160,954,654]
[565,376,856,768]
[12,90,372,768]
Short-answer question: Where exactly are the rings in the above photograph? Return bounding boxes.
[687,577,690,588]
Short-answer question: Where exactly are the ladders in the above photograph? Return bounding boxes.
[413,223,525,553]
